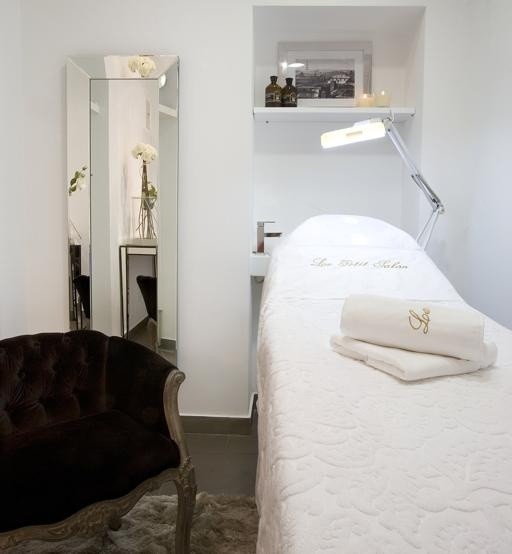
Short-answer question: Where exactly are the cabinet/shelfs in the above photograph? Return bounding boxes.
[250,102,419,280]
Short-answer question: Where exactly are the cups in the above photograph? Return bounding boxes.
[355,89,392,107]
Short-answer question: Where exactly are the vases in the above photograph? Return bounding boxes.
[131,195,157,238]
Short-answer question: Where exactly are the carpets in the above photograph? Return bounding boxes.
[2,487,260,552]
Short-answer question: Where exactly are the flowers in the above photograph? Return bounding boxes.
[129,141,158,239]
[68,161,88,198]
[126,55,158,77]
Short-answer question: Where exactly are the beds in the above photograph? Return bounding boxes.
[256,213,512,554]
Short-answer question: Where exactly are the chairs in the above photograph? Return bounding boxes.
[135,273,158,353]
[71,272,92,331]
[2,325,197,552]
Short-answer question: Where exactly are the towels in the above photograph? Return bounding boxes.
[336,296,482,362]
[331,331,498,384]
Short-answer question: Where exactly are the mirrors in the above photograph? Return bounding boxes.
[65,50,181,367]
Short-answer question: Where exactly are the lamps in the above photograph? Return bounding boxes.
[321,109,447,252]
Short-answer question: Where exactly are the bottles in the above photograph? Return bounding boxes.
[282,78,297,106]
[264,75,282,106]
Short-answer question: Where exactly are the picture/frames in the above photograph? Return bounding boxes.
[276,39,373,108]
